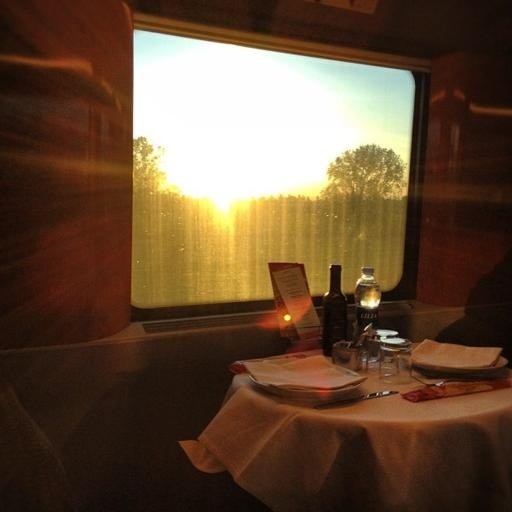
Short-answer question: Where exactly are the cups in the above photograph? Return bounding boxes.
[332,328,415,385]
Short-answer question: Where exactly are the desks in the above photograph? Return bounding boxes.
[225,340,512,511]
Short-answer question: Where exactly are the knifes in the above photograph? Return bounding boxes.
[314,391,397,410]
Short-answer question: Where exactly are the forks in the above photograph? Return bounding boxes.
[412,374,509,387]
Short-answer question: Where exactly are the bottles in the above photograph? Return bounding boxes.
[351,261,381,354]
[320,262,350,357]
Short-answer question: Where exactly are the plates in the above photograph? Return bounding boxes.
[248,371,361,401]
[409,340,508,373]
